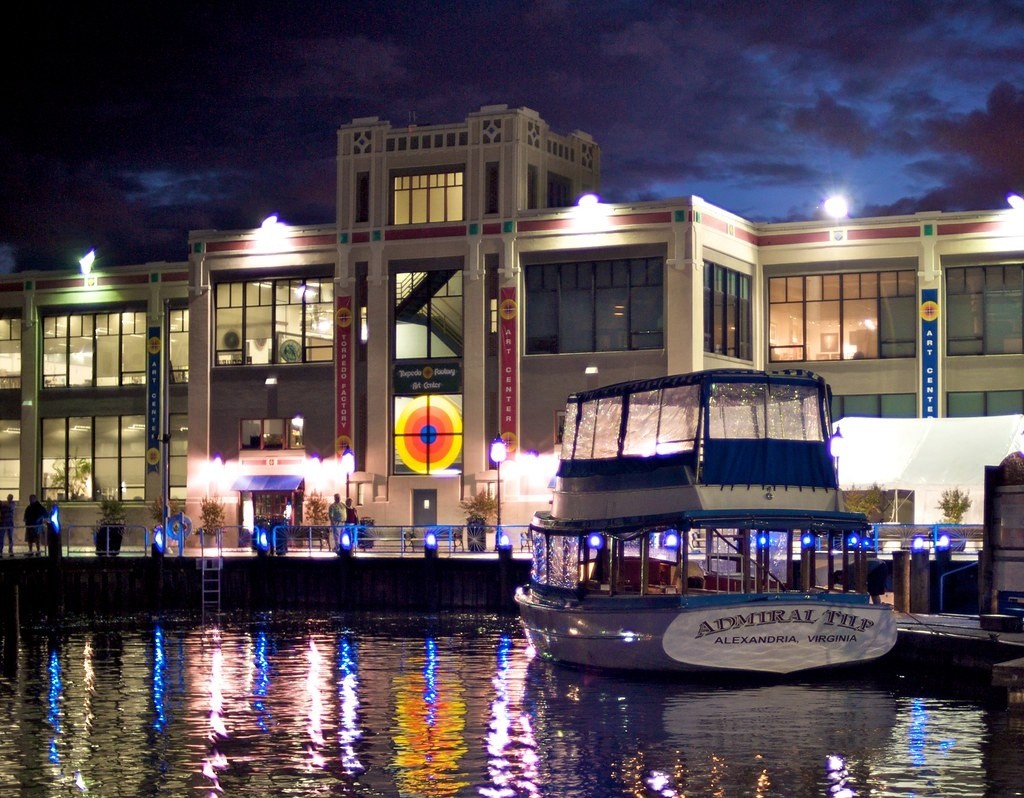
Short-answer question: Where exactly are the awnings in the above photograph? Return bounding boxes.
[229,475,304,491]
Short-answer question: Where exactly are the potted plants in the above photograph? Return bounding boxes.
[40,497,61,546]
[934,483,973,552]
[149,496,180,556]
[461,487,505,552]
[51,445,92,501]
[299,487,330,547]
[195,492,228,547]
[842,479,884,549]
[92,495,129,555]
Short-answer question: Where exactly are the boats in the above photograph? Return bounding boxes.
[513,369,897,679]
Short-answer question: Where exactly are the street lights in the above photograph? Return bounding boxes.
[341,446,355,500]
[488,433,506,553]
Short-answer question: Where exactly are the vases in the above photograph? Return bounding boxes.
[57,493,65,501]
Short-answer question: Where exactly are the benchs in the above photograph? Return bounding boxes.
[520,525,532,552]
[288,525,331,552]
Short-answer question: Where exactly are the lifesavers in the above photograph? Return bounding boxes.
[166,514,192,540]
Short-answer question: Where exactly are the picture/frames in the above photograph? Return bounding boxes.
[820,333,838,352]
[770,322,777,345]
[816,354,830,359]
[831,354,840,359]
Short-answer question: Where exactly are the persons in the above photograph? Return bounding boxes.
[0,494,17,558]
[284,504,292,527]
[329,493,359,551]
[23,494,49,556]
